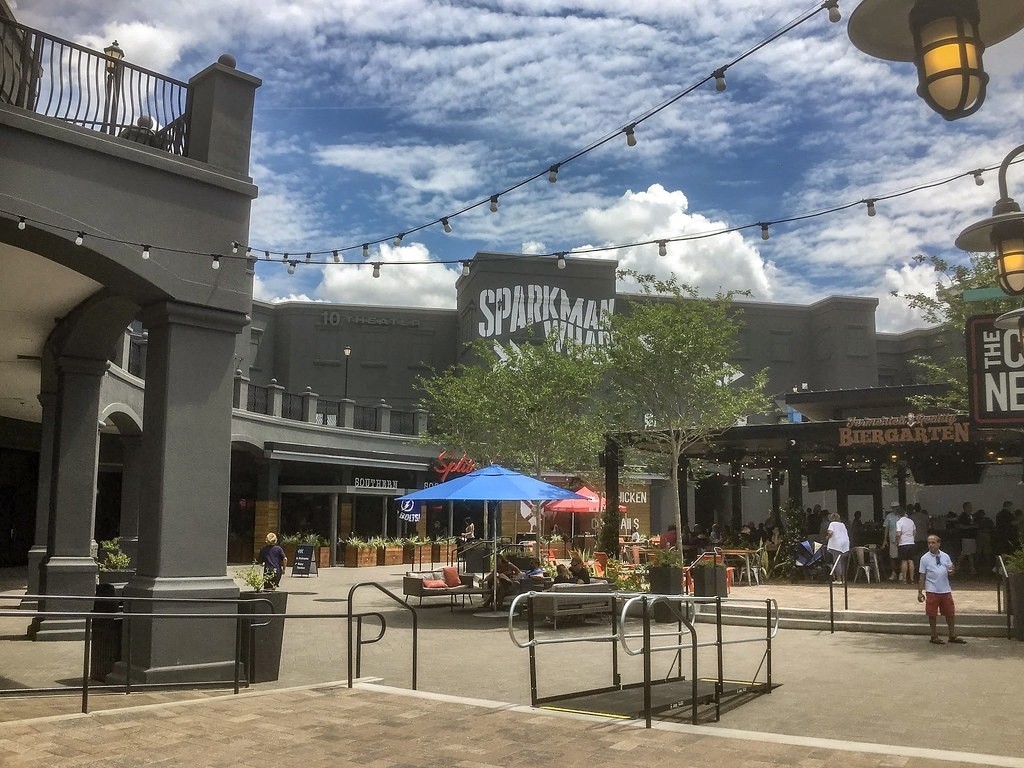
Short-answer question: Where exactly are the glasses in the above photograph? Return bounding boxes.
[936,556,940,565]
[570,562,580,567]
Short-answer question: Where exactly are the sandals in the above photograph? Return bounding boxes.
[929,637,946,644]
[947,635,967,644]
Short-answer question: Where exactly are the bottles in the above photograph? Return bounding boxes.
[948,562,953,572]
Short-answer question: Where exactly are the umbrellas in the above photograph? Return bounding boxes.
[395,464,593,617]
[796,541,824,569]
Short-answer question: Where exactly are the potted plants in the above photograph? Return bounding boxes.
[644,548,684,624]
[549,534,566,559]
[232,558,289,684]
[693,559,727,597]
[90,536,139,664]
[277,532,458,567]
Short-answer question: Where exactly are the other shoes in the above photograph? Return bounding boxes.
[832,580,845,585]
[899,573,904,581]
[889,573,898,580]
[968,569,982,576]
[477,604,489,608]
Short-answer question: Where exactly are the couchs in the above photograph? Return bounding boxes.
[403,569,474,608]
[483,571,552,616]
[544,577,609,630]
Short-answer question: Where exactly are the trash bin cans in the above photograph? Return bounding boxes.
[90,600,124,682]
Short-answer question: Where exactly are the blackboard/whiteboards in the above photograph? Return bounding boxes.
[292,546,318,575]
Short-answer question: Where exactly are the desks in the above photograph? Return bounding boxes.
[446,588,493,612]
[619,541,646,545]
[716,549,755,586]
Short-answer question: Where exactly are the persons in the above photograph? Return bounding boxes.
[947,501,1024,574]
[349,532,355,542]
[435,521,441,540]
[884,502,930,584]
[660,521,781,564]
[827,513,849,584]
[631,527,640,542]
[852,511,865,547]
[483,554,590,610]
[918,535,967,644]
[258,533,287,590]
[462,517,475,543]
[766,504,830,535]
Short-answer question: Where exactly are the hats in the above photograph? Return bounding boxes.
[265,532,277,546]
[890,501,899,507]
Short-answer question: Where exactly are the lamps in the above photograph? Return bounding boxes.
[846,0,1024,124]
[954,144,1024,297]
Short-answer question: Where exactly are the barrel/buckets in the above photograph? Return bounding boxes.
[282,567,293,576]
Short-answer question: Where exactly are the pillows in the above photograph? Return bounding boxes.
[422,579,448,588]
[442,566,462,587]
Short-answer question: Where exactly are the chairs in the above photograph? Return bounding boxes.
[569,514,950,595]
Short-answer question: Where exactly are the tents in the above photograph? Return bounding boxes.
[543,486,627,556]
